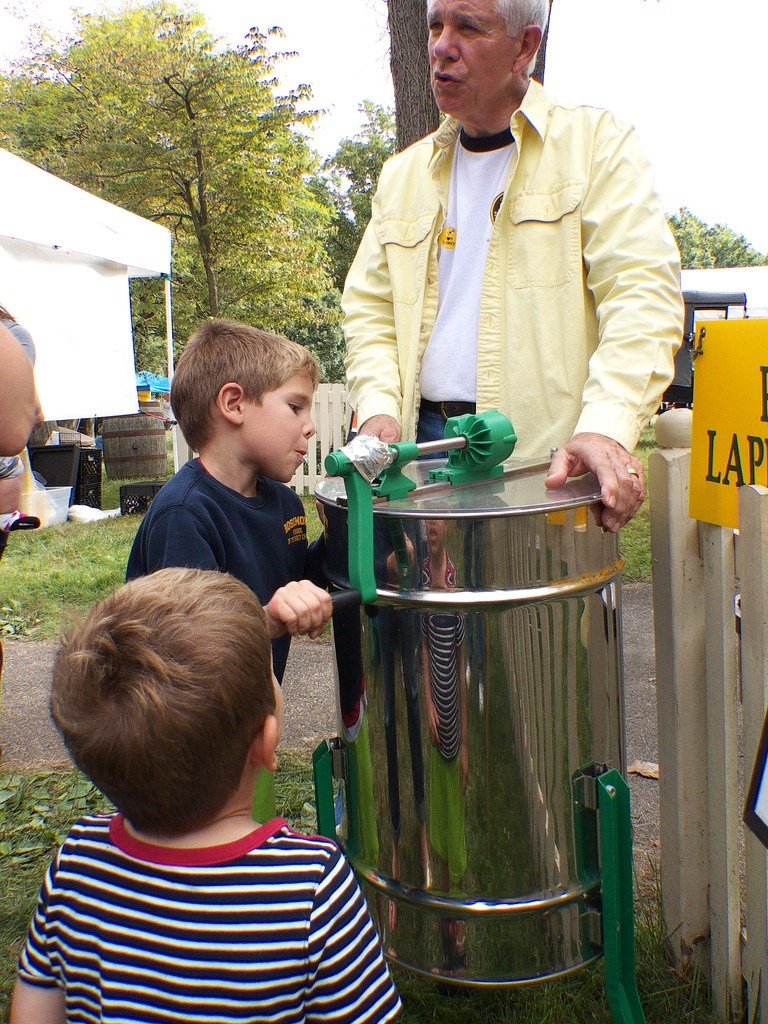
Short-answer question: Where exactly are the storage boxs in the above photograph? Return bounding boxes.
[74,449,102,510]
[120,481,167,516]
[18,486,73,532]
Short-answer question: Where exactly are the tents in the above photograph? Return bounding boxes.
[0,146,179,476]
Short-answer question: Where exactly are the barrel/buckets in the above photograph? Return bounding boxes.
[138,385,151,401]
[102,401,168,480]
[315,457,629,987]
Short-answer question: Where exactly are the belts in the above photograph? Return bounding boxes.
[418,397,476,422]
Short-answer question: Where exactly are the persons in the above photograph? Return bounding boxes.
[127,317,333,826]
[339,0,688,534]
[365,518,479,967]
[10,565,403,1024]
[0,303,44,675]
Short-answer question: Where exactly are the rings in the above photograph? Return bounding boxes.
[627,469,639,479]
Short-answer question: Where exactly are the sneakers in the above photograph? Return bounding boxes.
[440,917,467,970]
